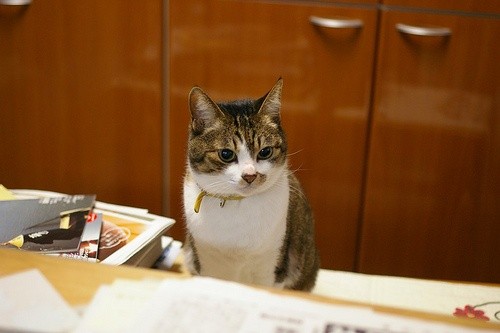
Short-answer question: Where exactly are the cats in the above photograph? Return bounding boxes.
[180,76,319,293]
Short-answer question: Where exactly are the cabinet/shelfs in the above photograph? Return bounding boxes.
[0,0,499,285]
